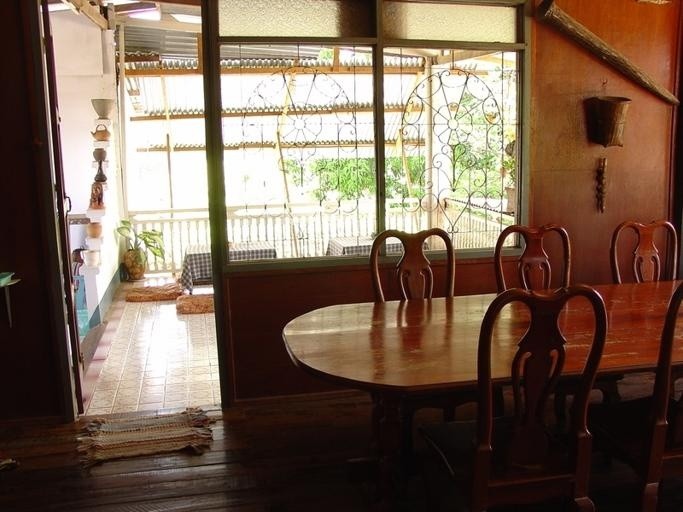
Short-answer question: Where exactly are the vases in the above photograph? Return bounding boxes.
[87,221,102,238]
[93,148,107,161]
[94,160,108,182]
[83,250,100,266]
[91,99,113,119]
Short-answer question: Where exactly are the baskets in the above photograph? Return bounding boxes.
[595,95,632,148]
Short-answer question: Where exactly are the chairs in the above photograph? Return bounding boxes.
[411,283,609,511]
[372,227,456,301]
[609,282,682,511]
[495,225,571,292]
[610,221,679,284]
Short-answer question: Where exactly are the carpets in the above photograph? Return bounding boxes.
[76,405,214,470]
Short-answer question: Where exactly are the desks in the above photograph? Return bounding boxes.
[325,235,430,257]
[180,240,277,294]
[280,279,683,511]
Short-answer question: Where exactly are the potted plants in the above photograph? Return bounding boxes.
[501,160,517,213]
[116,220,166,281]
